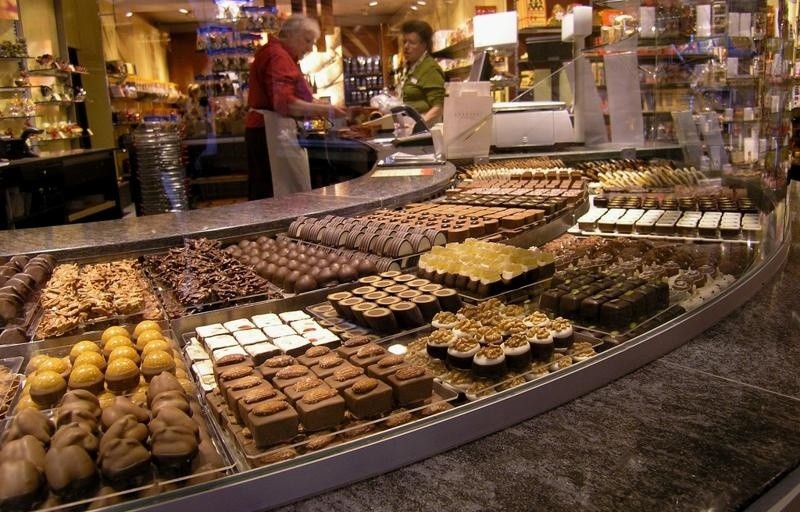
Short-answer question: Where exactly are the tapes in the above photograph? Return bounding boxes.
[368,111,383,121]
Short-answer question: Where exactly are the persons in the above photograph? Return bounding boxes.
[245,13,351,200]
[397,20,447,127]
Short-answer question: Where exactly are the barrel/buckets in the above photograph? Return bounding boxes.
[131,115,187,214]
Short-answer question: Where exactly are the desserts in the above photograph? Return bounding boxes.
[0,153,765,512]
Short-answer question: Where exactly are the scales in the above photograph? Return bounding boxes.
[489,6,593,153]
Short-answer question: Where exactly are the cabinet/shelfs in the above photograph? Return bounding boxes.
[0,52,94,145]
[109,71,184,155]
[427,10,800,182]
